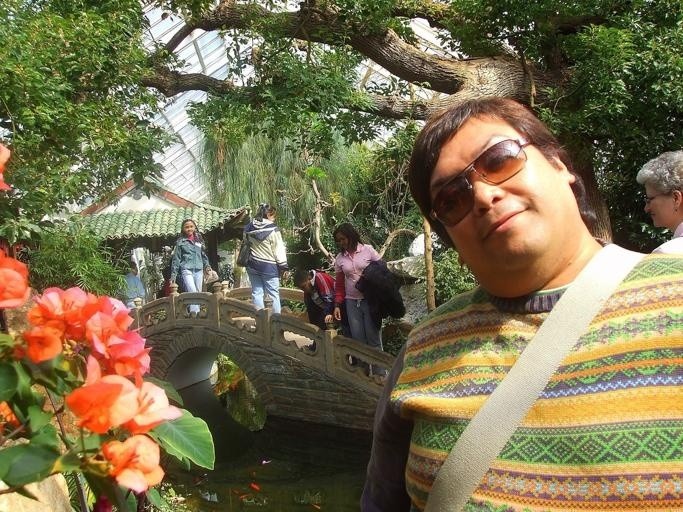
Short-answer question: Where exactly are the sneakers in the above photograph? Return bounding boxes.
[301,344,313,355]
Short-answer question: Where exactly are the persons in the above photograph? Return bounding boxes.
[358,96,682,512]
[125,261,146,308]
[162,266,172,296]
[295,269,348,336]
[170,219,211,318]
[333,222,385,375]
[634,150,683,260]
[243,204,289,314]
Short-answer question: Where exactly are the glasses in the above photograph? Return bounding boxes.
[643,193,663,204]
[428,139,534,227]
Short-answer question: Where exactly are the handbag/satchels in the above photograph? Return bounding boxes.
[203,267,219,285]
[236,233,250,267]
